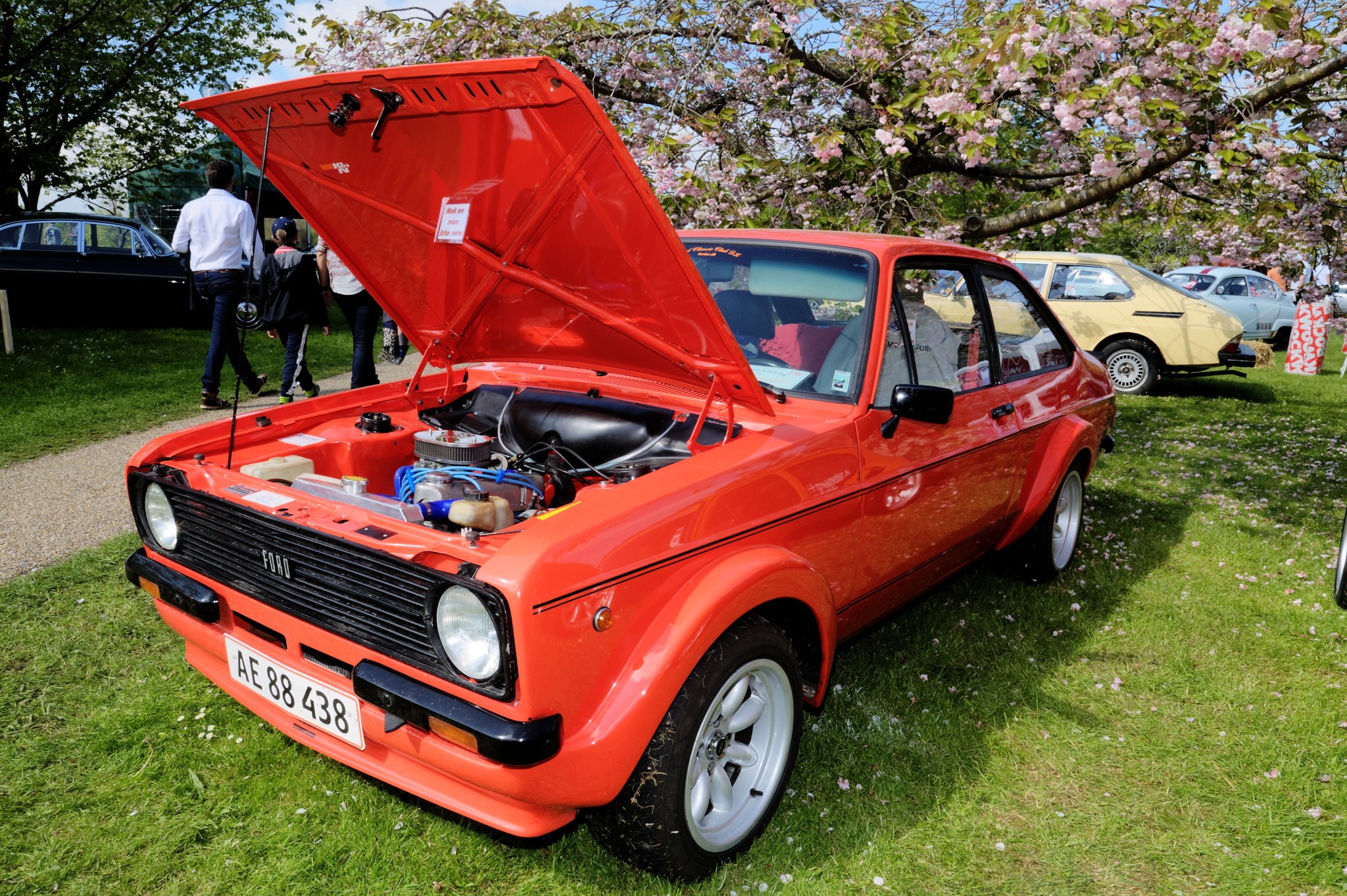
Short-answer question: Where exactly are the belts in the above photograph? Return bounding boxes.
[194,269,233,273]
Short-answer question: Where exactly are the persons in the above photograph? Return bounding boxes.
[172,160,267,409]
[1264,251,1333,306]
[316,231,380,389]
[44,222,87,246]
[255,217,330,404]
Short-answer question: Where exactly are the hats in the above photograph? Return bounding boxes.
[272,216,298,241]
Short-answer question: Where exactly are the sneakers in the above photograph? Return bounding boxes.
[199,391,228,408]
[279,396,293,404]
[304,383,320,398]
[247,373,267,396]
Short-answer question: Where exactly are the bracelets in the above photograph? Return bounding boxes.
[1297,287,1302,289]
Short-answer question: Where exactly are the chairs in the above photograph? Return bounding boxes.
[1190,282,1212,292]
[714,289,791,369]
[1228,284,1245,296]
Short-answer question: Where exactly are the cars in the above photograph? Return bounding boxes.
[1030,266,1130,296]
[1160,266,1297,351]
[819,250,1256,396]
[1284,279,1347,317]
[118,54,1120,888]
[0,211,266,333]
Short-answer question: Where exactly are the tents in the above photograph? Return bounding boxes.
[127,135,313,249]
